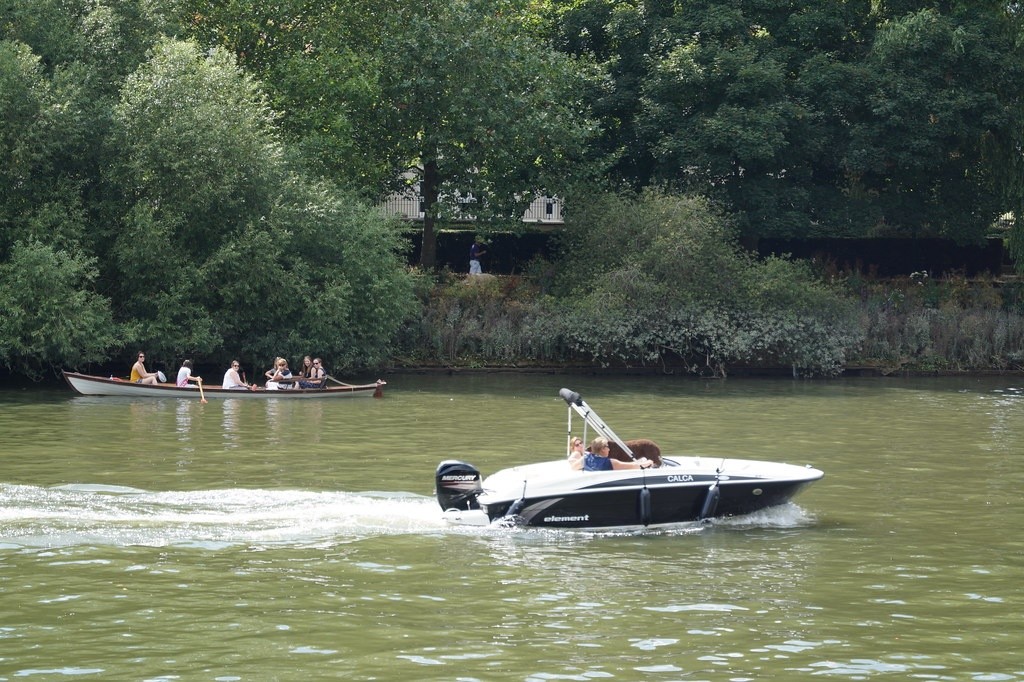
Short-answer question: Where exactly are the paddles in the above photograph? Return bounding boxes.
[243,372,246,384]
[247,384,256,392]
[199,379,206,400]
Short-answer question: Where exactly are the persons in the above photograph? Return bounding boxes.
[572,437,654,472]
[266,359,292,388]
[265,357,281,378]
[177,359,202,388]
[130,351,158,384]
[469,242,486,274]
[222,360,246,389]
[294,355,326,389]
[569,437,584,464]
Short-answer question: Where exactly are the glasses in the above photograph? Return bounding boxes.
[604,445,612,449]
[278,364,285,367]
[139,356,145,358]
[575,441,583,448]
[233,365,240,368]
[313,362,319,365]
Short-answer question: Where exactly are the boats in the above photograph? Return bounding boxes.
[434,383,826,532]
[61,365,387,399]
